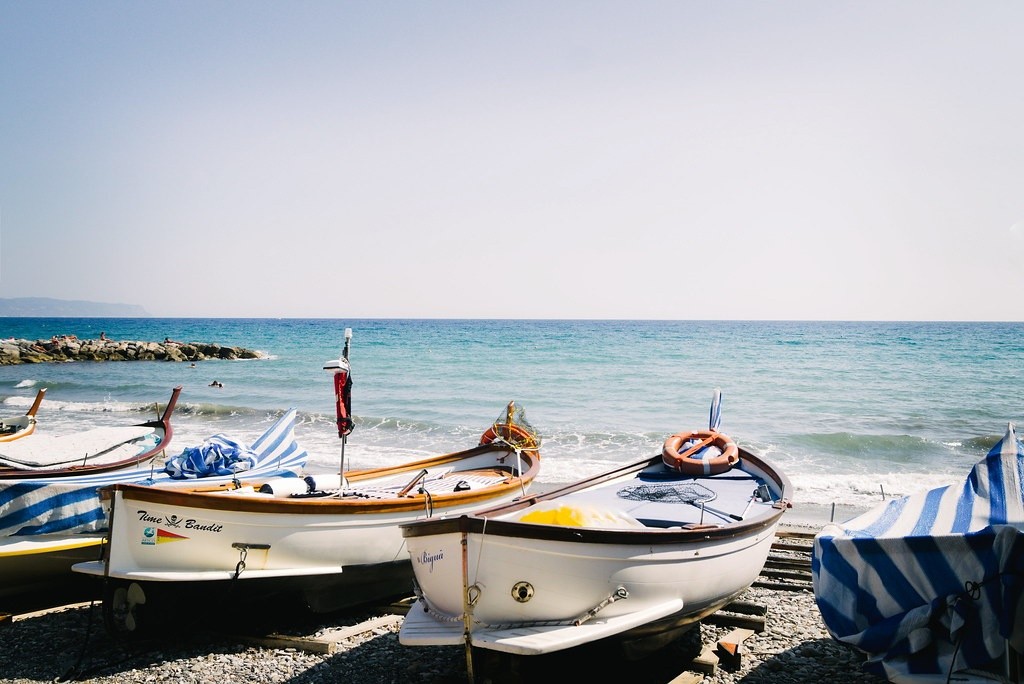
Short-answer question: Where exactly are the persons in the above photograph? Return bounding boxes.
[101,332,113,343]
[165,337,172,343]
[52,335,58,346]
[65,334,77,340]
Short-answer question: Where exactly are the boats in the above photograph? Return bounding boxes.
[399,386,794,658]
[0,386,304,598]
[811,423,1024,684]
[69,403,542,643]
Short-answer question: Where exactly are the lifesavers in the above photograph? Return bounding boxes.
[479,422,540,461]
[662,429,739,475]
